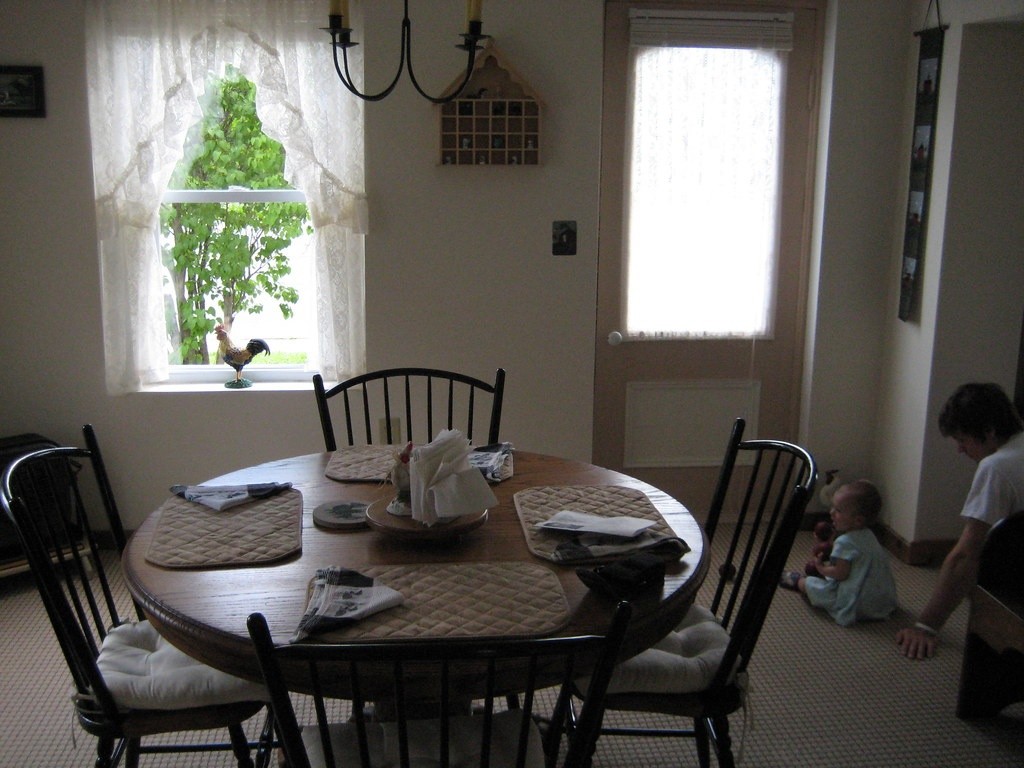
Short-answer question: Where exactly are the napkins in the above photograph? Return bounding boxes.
[409,427,512,528]
[549,528,692,569]
[167,482,294,512]
[287,562,404,642]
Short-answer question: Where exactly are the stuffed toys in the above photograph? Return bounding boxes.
[804,521,833,579]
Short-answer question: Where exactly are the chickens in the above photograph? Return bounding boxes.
[214,323,271,380]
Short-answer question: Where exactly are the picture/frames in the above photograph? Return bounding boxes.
[0,65,49,118]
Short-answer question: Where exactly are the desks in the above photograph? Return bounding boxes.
[121,449,712,723]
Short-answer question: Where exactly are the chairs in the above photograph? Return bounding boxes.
[0,423,274,768]
[544,416,817,768]
[313,367,506,448]
[245,599,632,768]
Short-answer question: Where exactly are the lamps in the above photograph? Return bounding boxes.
[318,0,492,104]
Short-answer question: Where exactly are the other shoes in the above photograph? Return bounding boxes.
[575,557,667,596]
[777,571,801,589]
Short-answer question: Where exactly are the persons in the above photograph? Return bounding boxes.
[779,480,900,629]
[895,382,1024,661]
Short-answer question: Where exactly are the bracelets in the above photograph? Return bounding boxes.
[912,622,941,639]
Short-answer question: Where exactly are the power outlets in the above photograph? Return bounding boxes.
[378,417,401,444]
